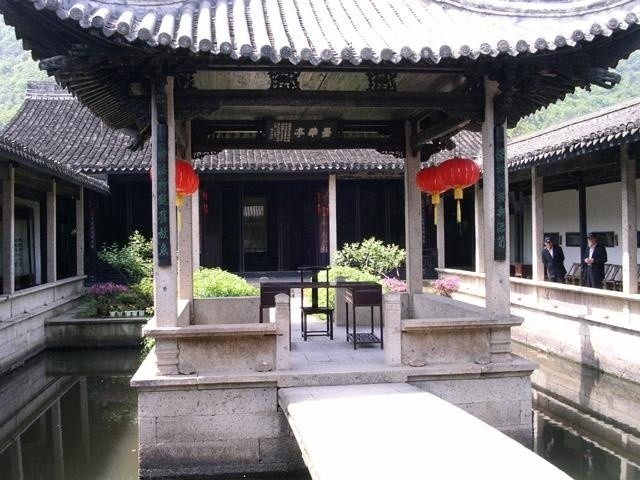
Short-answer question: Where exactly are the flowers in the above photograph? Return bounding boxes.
[91,282,128,307]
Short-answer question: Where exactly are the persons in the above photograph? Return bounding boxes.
[540,421,566,459]
[542,236,567,283]
[582,444,605,480]
[581,232,607,288]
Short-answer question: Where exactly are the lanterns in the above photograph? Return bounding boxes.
[437,151,480,224]
[150,159,201,235]
[416,162,449,226]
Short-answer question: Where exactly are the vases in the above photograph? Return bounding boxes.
[98,307,110,318]
[109,310,146,318]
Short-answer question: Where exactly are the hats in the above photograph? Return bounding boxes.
[587,232,601,239]
[544,235,553,243]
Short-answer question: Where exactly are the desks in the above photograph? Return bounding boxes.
[510,261,532,279]
[257,279,384,352]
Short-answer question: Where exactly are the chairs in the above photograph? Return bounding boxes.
[298,265,336,342]
[562,261,640,294]
